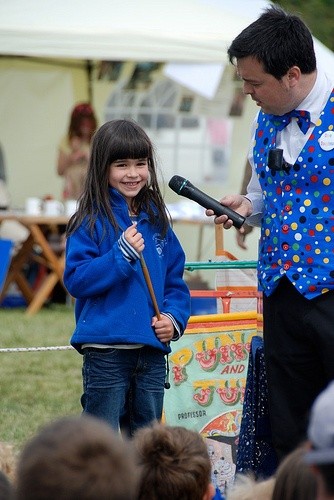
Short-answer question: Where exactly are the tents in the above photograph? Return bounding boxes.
[0,0,334,293]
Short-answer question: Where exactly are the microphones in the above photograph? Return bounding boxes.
[168,175,246,230]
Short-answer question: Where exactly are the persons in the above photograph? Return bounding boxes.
[58,103,97,199]
[63,120,191,439]
[0,377,334,500]
[205,3,334,478]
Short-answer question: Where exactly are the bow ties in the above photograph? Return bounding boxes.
[270,110,311,134]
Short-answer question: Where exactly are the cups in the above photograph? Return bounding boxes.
[43,201,63,217]
[66,201,76,217]
[26,197,41,215]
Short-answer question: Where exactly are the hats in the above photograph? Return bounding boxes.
[294,381,334,463]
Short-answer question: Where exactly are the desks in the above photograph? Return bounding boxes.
[0,213,219,316]
